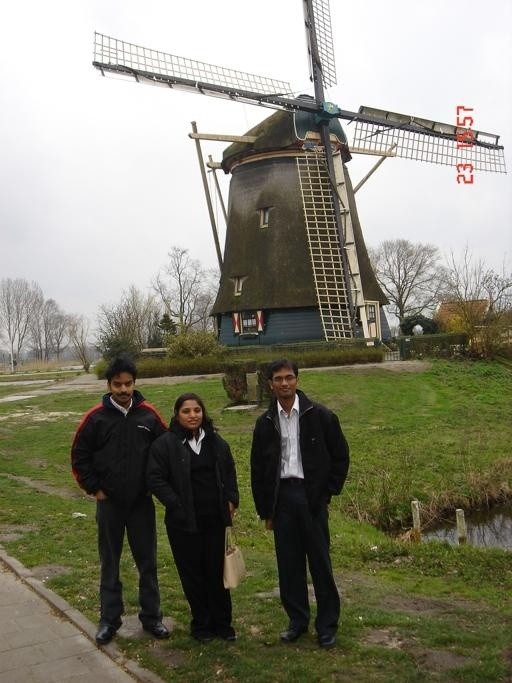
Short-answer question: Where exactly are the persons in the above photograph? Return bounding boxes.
[251,353,347,648]
[70,352,169,646]
[144,391,242,646]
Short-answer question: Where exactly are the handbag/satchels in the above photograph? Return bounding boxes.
[222,526,247,589]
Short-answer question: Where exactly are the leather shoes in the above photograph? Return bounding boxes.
[220,631,236,642]
[143,621,170,638]
[95,625,117,645]
[279,627,308,642]
[195,634,216,642]
[318,633,337,649]
